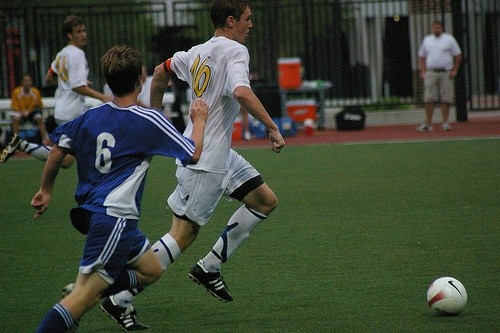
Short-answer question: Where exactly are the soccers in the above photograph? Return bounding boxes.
[427,275,468,316]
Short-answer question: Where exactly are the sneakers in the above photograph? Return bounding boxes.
[443,124,451,132]
[100,293,149,332]
[0,134,22,164]
[416,123,432,132]
[188,259,235,304]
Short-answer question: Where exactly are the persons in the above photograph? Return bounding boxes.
[102,63,198,137]
[63,0,287,303]
[0,15,114,168]
[415,21,462,132]
[9,75,52,146]
[31,42,210,333]
[240,106,252,141]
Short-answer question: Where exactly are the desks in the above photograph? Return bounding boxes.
[280,80,333,129]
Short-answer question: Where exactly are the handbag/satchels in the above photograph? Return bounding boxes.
[335,106,366,131]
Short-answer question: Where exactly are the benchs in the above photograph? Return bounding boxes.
[0,97,55,132]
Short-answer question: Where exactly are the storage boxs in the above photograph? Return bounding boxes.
[286,98,319,123]
[232,117,242,140]
[278,57,303,90]
